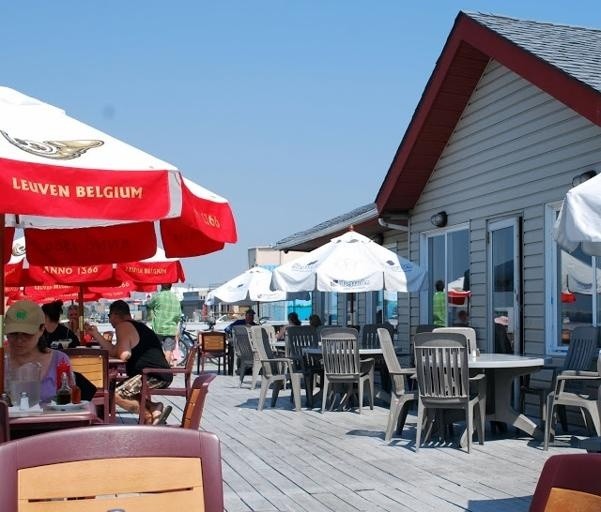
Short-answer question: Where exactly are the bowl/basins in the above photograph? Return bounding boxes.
[62,342,70,349]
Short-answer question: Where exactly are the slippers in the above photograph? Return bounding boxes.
[153,406,172,426]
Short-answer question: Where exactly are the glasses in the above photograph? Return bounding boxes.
[108,313,115,317]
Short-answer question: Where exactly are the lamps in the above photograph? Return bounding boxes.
[366,231,385,245]
[427,210,449,230]
[567,166,597,190]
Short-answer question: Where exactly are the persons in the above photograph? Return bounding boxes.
[61,305,91,342]
[4,301,76,406]
[276,313,301,341]
[432,279,447,329]
[224,309,259,335]
[38,299,79,354]
[308,314,322,327]
[146,282,182,364]
[86,301,174,424]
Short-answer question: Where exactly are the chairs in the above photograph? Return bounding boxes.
[178,367,218,430]
[432,324,482,357]
[548,324,596,440]
[376,322,417,446]
[412,331,488,453]
[539,347,601,446]
[363,321,396,350]
[52,349,110,423]
[286,327,322,408]
[232,325,255,387]
[316,325,376,417]
[243,324,298,412]
[137,335,199,426]
[196,330,234,376]
[530,452,601,512]
[0,418,225,509]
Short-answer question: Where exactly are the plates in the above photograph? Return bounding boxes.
[48,400,91,411]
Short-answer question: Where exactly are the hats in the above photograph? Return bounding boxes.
[246,309,256,314]
[3,300,46,335]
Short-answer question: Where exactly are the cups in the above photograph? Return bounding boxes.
[71,385,81,404]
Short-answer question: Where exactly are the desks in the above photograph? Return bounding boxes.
[304,343,387,415]
[407,352,555,448]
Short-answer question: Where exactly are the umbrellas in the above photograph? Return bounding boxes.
[0,236,186,345]
[270,231,425,326]
[5,285,170,340]
[561,247,601,303]
[204,266,311,321]
[0,85,238,404]
[550,172,601,256]
[447,276,471,317]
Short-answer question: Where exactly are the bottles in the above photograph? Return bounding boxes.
[20,392,29,410]
[55,357,70,390]
[57,372,71,404]
[473,358,476,363]
[1,393,11,407]
[472,348,480,358]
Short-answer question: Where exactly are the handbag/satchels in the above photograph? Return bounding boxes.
[171,348,180,360]
